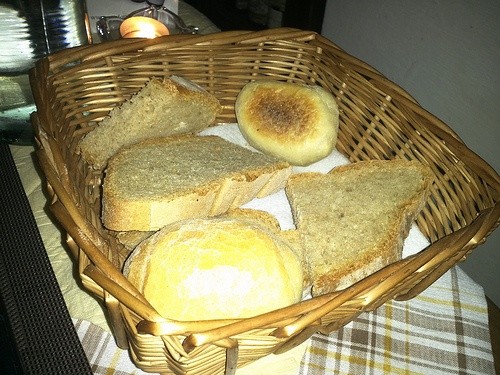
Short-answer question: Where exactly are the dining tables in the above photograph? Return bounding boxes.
[0,103,500,374]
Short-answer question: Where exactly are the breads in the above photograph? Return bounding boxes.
[235,81,340,167]
[122,209,312,323]
[101,133,292,232]
[285,159,435,299]
[74,75,222,170]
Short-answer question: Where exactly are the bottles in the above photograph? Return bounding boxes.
[39,0,93,50]
[0,0,49,75]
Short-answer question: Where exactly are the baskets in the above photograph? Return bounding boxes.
[29,28,500,375]
